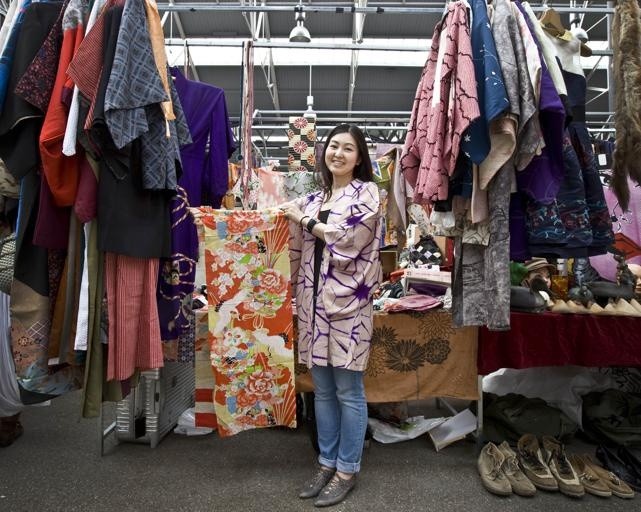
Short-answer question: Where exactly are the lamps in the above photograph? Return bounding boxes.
[302,66,316,121]
[571,11,589,44]
[289,5,311,43]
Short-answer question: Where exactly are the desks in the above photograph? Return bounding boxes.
[292,311,482,450]
[479,308,640,430]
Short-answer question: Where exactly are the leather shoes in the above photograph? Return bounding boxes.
[298,464,338,499]
[312,470,357,508]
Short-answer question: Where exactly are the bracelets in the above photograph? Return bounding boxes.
[307,217,322,233]
[299,215,310,226]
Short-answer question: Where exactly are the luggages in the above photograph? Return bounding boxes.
[116,358,195,447]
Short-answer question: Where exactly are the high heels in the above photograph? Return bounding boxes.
[594,444,641,492]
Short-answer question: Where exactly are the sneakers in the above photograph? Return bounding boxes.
[551,296,641,316]
[476,440,538,498]
[567,451,636,500]
[0,407,26,450]
[516,431,586,498]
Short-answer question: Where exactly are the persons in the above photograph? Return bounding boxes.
[185,122,385,509]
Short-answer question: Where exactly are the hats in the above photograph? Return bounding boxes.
[522,256,557,273]
